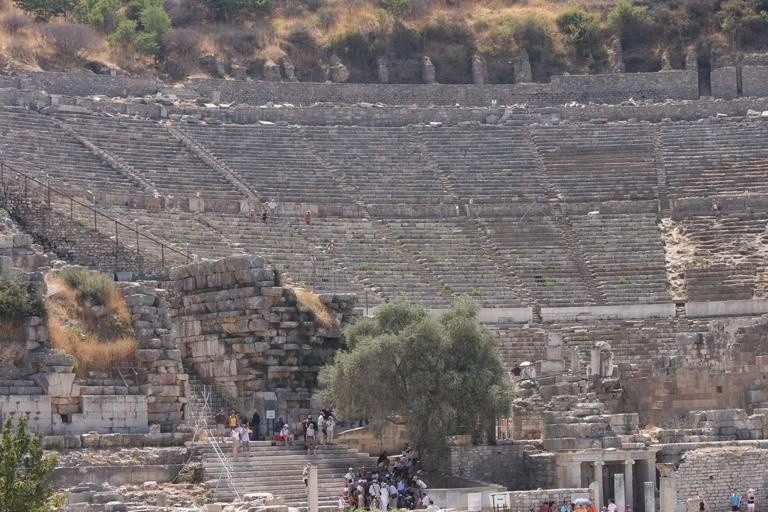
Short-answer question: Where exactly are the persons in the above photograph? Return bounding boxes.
[214,407,336,461]
[267,197,277,216]
[730,487,743,511]
[336,442,443,512]
[261,210,269,223]
[529,496,636,512]
[305,209,313,225]
[745,487,758,511]
[247,208,257,224]
[710,198,718,212]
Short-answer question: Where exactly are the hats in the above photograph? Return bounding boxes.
[308,415,312,418]
[284,424,288,427]
[745,489,755,493]
[359,480,386,487]
[412,476,420,480]
